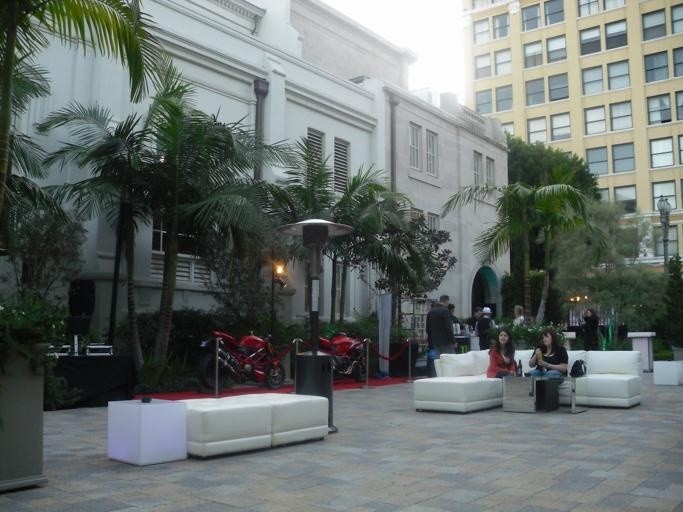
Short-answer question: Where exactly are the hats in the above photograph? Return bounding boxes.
[483,307,492,313]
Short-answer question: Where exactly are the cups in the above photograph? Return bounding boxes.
[141,397,150,402]
[524,373,531,377]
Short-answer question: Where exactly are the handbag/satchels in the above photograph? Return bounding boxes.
[570,360,586,377]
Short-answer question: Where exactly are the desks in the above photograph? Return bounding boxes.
[107,398,186,466]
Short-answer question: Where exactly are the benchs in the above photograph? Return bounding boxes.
[174,391,329,459]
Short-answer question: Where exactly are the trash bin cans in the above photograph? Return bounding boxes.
[46,343,133,408]
[456,340,467,354]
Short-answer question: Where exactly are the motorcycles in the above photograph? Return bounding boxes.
[189,328,290,392]
[315,331,369,381]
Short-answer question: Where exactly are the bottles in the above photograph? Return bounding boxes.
[516,360,522,376]
[451,320,474,353]
[535,348,541,361]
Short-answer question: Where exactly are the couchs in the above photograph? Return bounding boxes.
[414,349,642,414]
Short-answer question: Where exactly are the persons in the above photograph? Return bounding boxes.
[467,306,480,331]
[511,305,526,330]
[475,306,496,349]
[447,303,466,330]
[528,330,568,384]
[426,294,458,359]
[486,328,517,378]
[583,309,598,350]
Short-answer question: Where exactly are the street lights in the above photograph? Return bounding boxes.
[655,195,670,274]
